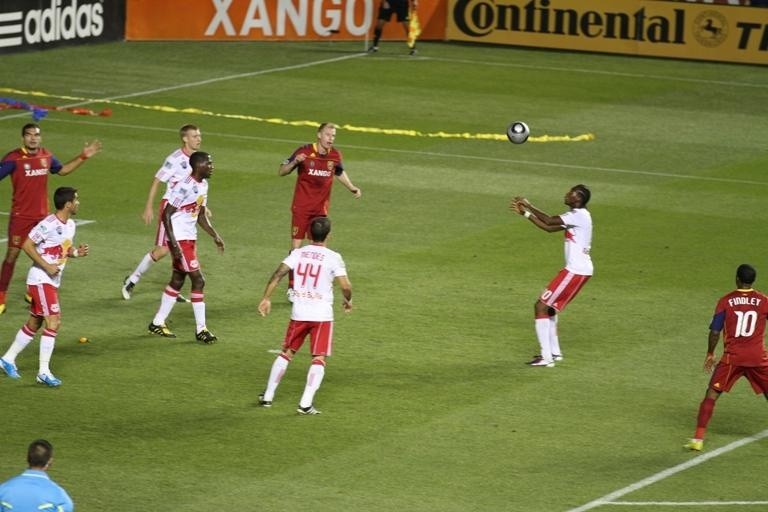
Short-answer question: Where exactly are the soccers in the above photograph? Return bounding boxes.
[508,121,530,144]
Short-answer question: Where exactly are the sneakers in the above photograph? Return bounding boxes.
[122,275,135,300]
[296,404,323,415]
[683,439,703,451]
[0,357,21,378]
[195,327,217,344]
[24,293,33,303]
[176,293,191,303]
[149,321,177,338]
[257,391,272,408]
[525,353,563,367]
[36,372,62,387]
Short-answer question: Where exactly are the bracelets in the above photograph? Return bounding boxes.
[523,211,531,218]
[73,248,79,258]
[343,296,352,306]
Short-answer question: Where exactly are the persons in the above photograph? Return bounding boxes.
[144,151,227,344]
[0,186,89,387]
[280,122,361,303]
[258,217,352,416]
[682,263,768,451]
[0,439,76,512]
[121,125,214,301]
[0,123,104,314]
[508,183,594,368]
[366,0,425,57]
